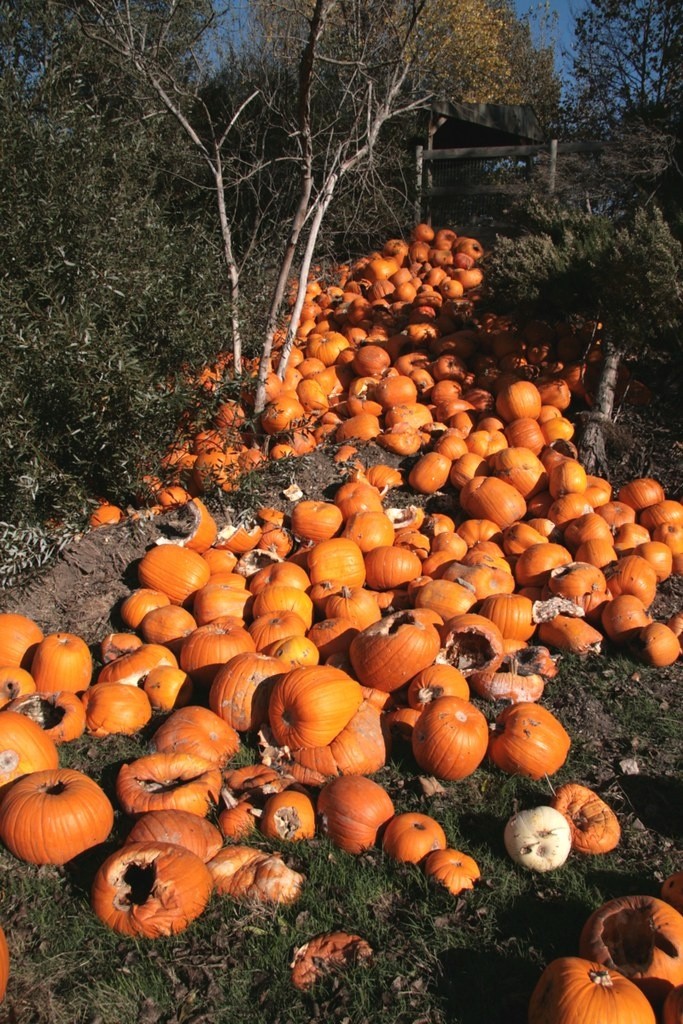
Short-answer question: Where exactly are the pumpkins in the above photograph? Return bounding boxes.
[0,224,683,1024]
